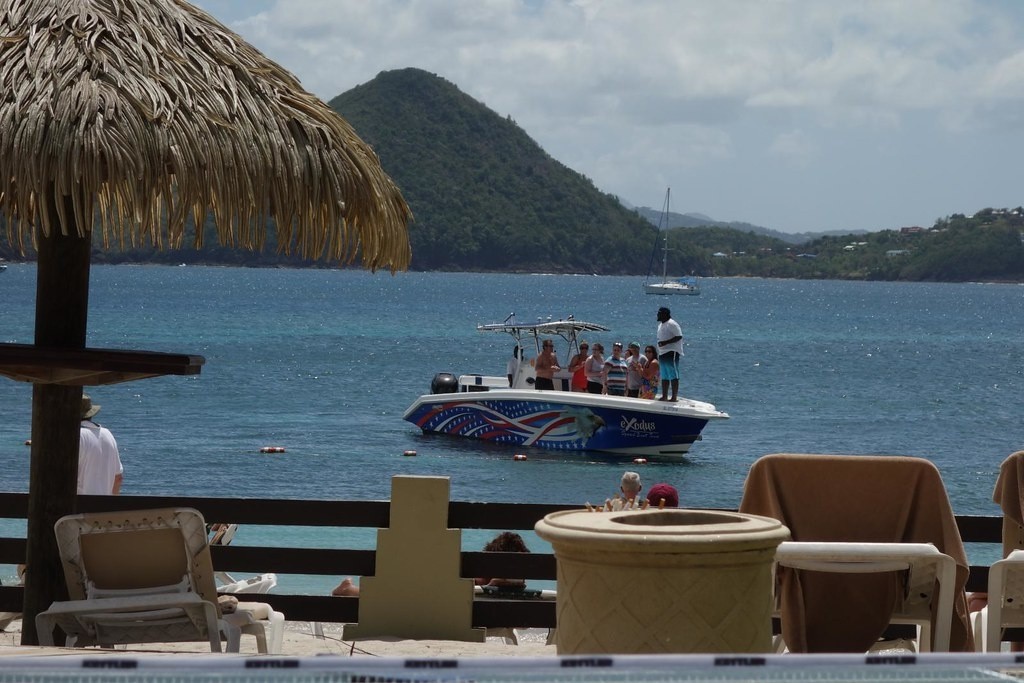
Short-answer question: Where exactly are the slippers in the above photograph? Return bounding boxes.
[659,397,668,401]
[669,399,678,402]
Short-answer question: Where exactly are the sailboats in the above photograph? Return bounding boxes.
[642,185,702,296]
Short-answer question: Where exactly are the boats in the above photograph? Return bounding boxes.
[402,310,732,462]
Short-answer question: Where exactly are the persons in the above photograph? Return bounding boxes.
[25,395,125,495]
[601,343,629,396]
[620,472,644,508]
[331,532,531,595]
[507,345,524,389]
[657,307,684,402]
[637,346,660,400]
[584,343,605,394]
[626,342,648,398]
[623,349,633,363]
[966,590,1024,651]
[568,343,589,393]
[533,339,561,391]
[648,484,678,508]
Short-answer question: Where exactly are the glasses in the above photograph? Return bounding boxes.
[613,343,623,347]
[645,350,652,353]
[592,349,599,351]
[581,347,588,350]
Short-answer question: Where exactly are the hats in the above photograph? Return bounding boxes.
[81,393,101,418]
[628,342,641,349]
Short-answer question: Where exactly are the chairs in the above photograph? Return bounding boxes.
[34,508,284,658]
[740,453,978,654]
[471,585,556,647]
[964,450,1024,653]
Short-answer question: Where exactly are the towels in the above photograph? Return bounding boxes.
[992,451,1024,661]
[735,450,977,657]
